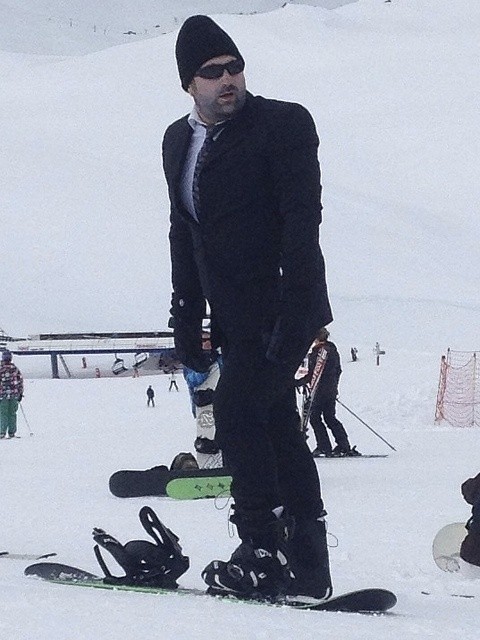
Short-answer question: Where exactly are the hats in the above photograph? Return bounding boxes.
[3,349,12,358]
[176,15,245,89]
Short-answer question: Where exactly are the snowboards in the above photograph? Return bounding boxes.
[25,563,396,611]
[166,476,235,500]
[109,468,233,498]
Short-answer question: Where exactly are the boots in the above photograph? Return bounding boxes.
[201,512,275,595]
[212,510,331,598]
[313,440,333,454]
[335,439,352,456]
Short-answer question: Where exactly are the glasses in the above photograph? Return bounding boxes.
[201,338,211,342]
[189,60,246,78]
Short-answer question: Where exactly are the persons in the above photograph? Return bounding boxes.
[132,363,141,378]
[293,327,351,454]
[168,371,179,393]
[0,348,24,439]
[146,385,155,407]
[161,13,335,609]
[354,348,358,354]
[351,348,356,361]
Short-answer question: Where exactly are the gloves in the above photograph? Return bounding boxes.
[173,323,222,372]
[262,316,305,365]
[18,394,24,402]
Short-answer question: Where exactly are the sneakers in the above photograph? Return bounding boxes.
[0,433,5,438]
[10,432,14,437]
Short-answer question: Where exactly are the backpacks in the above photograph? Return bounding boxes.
[169,452,199,471]
